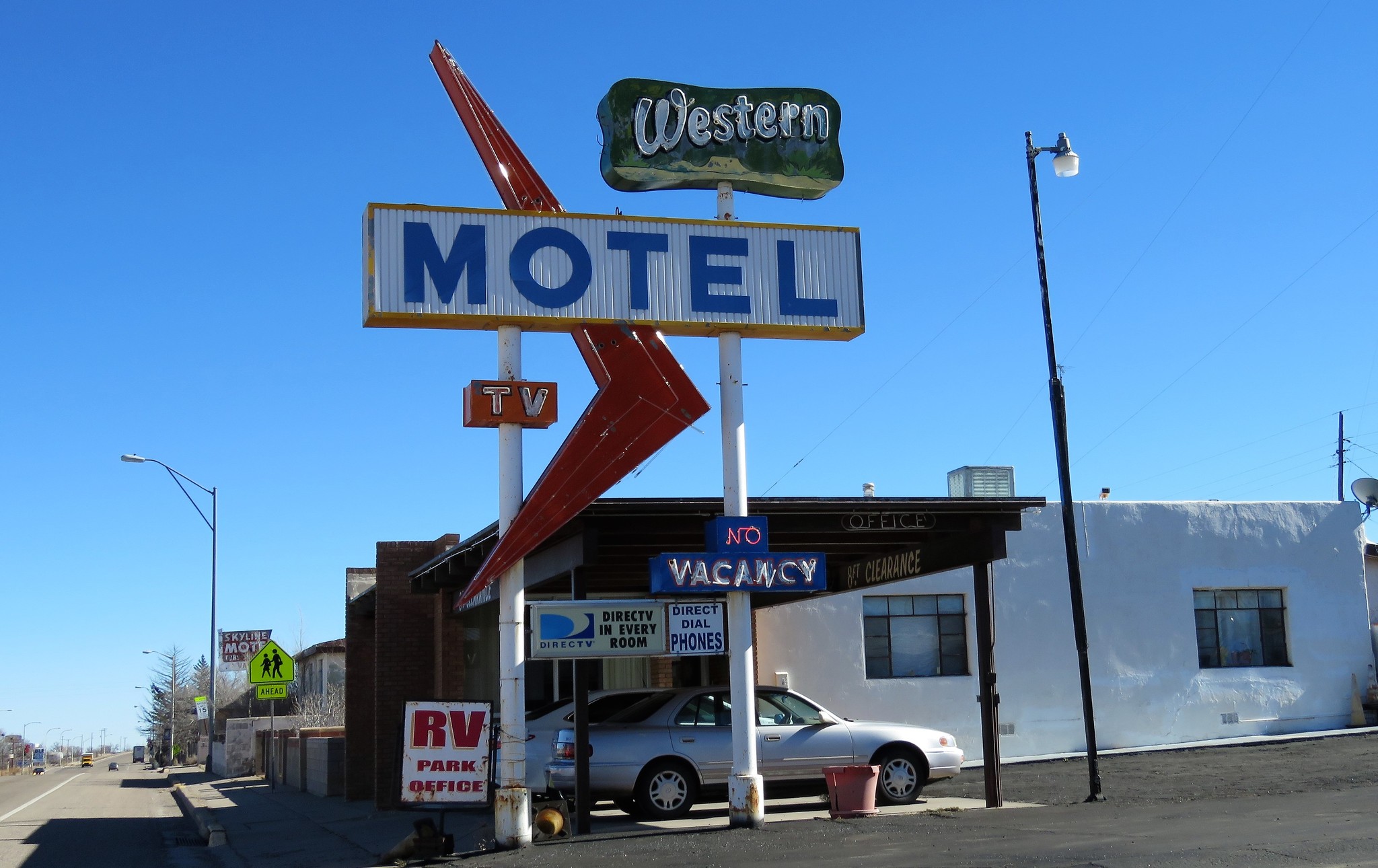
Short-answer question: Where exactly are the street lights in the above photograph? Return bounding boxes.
[45,727,61,769]
[10,737,14,767]
[21,722,42,775]
[1026,129,1108,803]
[120,453,217,774]
[1,734,5,776]
[142,650,176,765]
[59,729,73,767]
[134,705,155,764]
[135,686,162,765]
[72,732,112,766]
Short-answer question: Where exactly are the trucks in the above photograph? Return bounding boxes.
[133,746,145,763]
[80,752,94,768]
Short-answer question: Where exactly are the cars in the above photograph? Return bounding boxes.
[108,762,119,771]
[489,687,794,814]
[32,767,45,776]
[543,684,965,820]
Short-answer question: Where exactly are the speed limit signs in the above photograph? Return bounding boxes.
[194,699,210,720]
